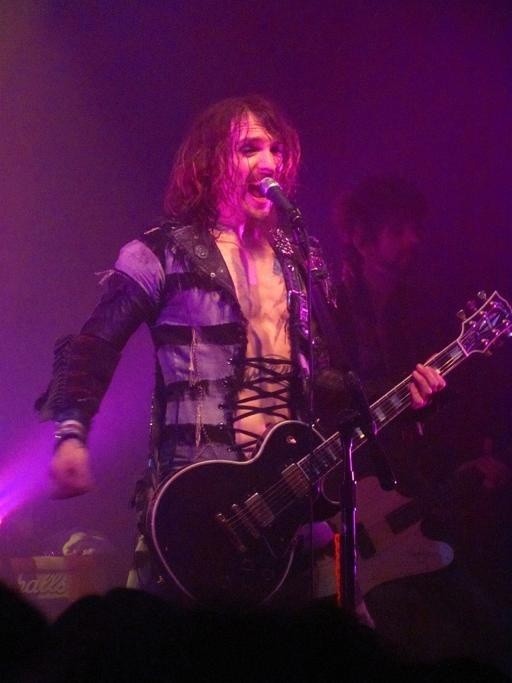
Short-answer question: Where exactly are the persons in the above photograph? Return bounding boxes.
[321,167,509,657]
[35,91,448,635]
[0,577,512,682]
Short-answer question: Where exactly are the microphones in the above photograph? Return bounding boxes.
[258,176,302,221]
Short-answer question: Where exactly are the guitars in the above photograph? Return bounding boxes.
[144,289,512,613]
[288,453,512,608]
